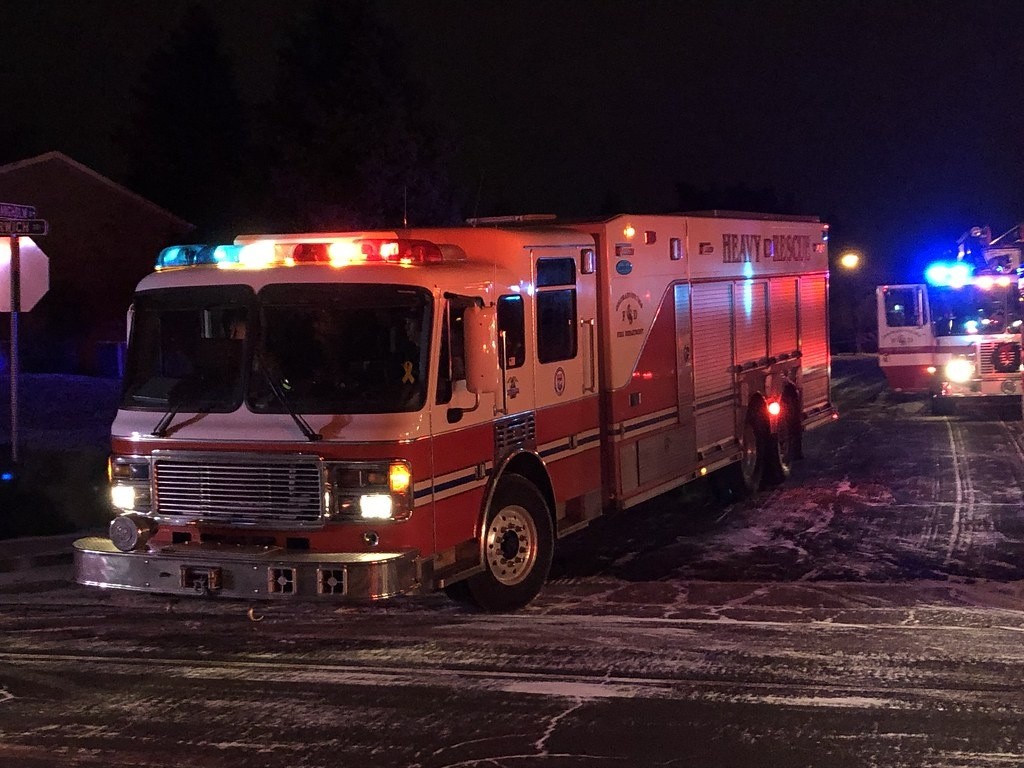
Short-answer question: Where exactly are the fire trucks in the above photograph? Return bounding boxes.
[73,214,839,614]
[876,224,1024,415]
